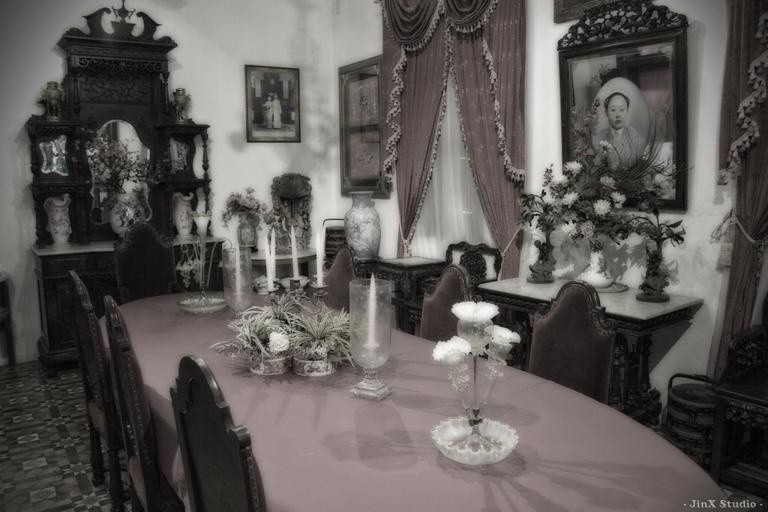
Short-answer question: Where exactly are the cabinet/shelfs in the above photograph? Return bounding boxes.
[152,236,229,293]
[480,267,703,424]
[32,238,122,372]
[27,2,215,244]
[376,255,447,330]
[719,328,767,498]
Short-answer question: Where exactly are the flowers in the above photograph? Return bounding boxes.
[617,173,686,264]
[565,156,620,255]
[223,190,267,227]
[221,296,291,357]
[89,142,128,225]
[515,160,596,238]
[120,151,146,192]
[428,297,522,422]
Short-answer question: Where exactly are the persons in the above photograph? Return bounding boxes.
[595,91,647,171]
[271,95,283,129]
[262,96,274,130]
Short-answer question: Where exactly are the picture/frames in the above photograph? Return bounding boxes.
[558,2,690,216]
[338,54,392,199]
[244,63,300,143]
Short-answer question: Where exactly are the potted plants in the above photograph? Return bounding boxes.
[293,305,348,377]
[270,167,311,254]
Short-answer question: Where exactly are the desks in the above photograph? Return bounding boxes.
[104,288,734,512]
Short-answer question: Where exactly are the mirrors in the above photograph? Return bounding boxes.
[84,118,153,225]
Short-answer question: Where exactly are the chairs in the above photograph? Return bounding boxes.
[170,344,268,509]
[416,264,475,344]
[59,265,125,510]
[100,288,177,511]
[526,277,619,406]
[392,241,501,335]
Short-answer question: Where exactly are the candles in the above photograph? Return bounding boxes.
[315,232,325,291]
[366,269,379,345]
[290,224,303,282]
[261,227,277,291]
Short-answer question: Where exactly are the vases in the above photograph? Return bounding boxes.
[343,195,381,265]
[527,231,558,287]
[576,252,614,288]
[237,224,263,252]
[637,268,674,305]
[113,184,153,237]
[42,194,76,246]
[434,360,519,467]
[252,350,291,374]
[170,193,201,241]
